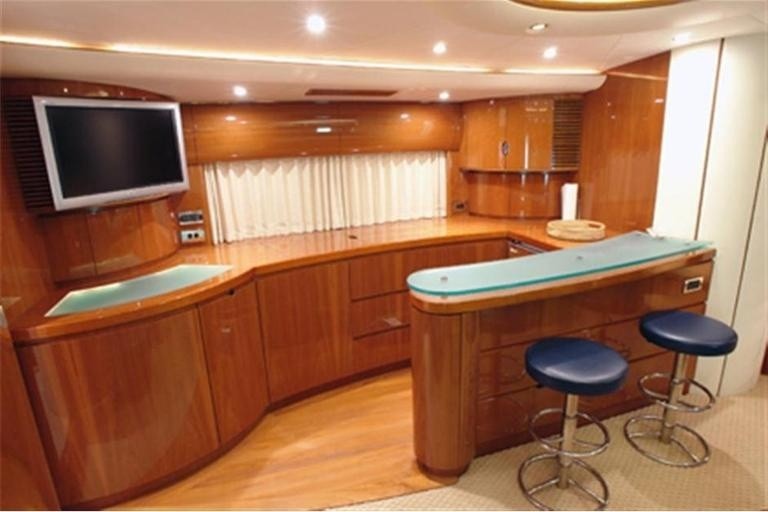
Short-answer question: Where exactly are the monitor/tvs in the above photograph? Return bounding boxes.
[31,95,189,211]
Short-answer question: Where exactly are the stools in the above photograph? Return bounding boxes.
[516,337,628,511]
[624,310,737,467]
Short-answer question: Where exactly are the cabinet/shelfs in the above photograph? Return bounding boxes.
[260,233,512,407]
[197,105,461,151]
[467,108,559,213]
[36,278,260,510]
[53,197,181,290]
